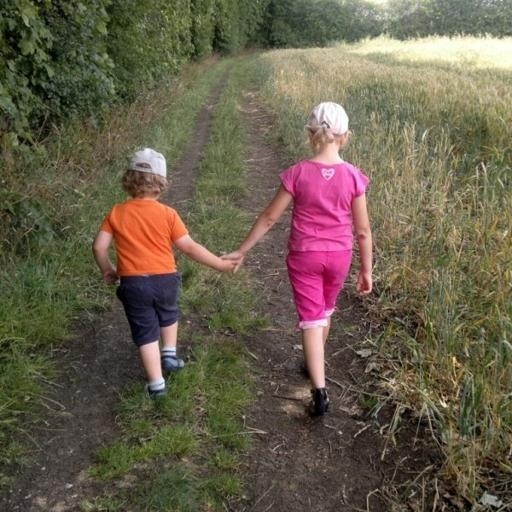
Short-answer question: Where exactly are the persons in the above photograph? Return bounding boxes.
[93,148,238,405]
[220,101,375,420]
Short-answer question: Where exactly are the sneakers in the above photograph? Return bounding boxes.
[309,388,330,415]
[146,381,168,401]
[160,355,185,371]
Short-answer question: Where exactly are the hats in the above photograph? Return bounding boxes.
[125,147,169,181]
[303,101,349,139]
